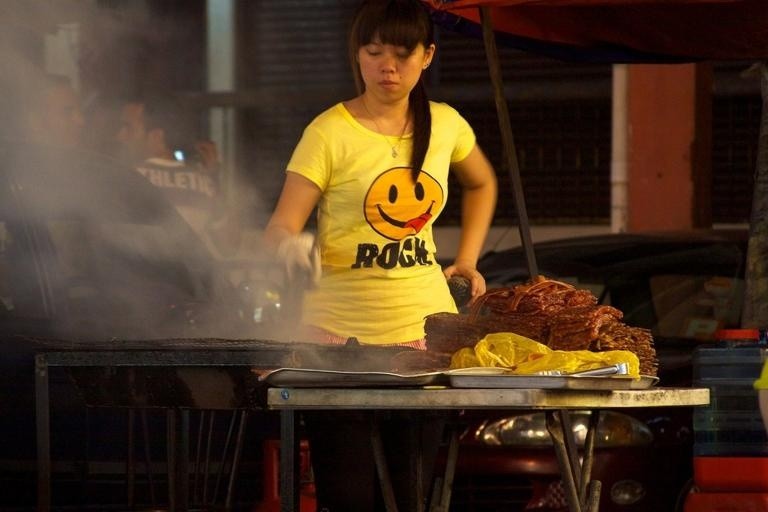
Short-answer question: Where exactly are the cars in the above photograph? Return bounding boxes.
[437,227,748,332]
[0,137,250,504]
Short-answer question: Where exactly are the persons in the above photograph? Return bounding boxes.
[109,87,223,248]
[2,72,84,150]
[264,1,501,511]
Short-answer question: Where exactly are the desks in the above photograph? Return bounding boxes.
[266,385,711,511]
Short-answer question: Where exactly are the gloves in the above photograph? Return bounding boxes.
[278,235,321,284]
